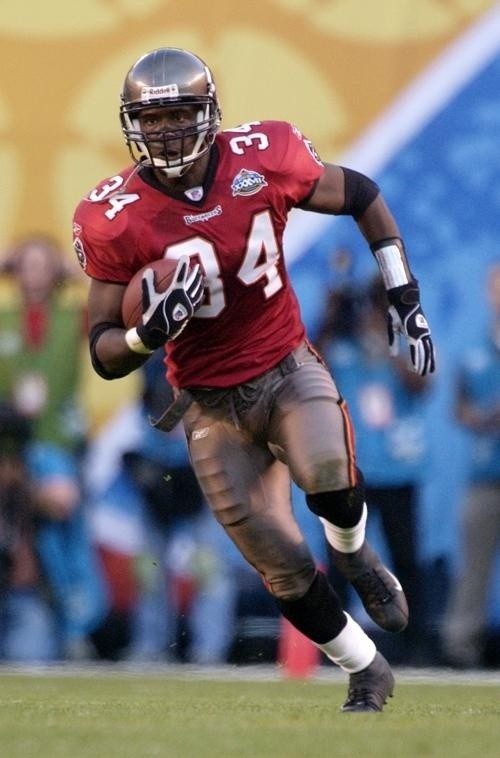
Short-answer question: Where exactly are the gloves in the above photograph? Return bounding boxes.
[386,282,435,375]
[124,256,204,354]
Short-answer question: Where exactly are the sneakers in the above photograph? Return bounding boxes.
[325,540,409,633]
[342,651,395,712]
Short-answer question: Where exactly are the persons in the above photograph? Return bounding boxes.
[66,46,436,713]
[0,233,236,664]
[318,266,500,674]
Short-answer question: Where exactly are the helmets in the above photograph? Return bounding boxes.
[120,47,221,179]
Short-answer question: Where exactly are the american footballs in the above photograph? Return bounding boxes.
[121,258,193,330]
